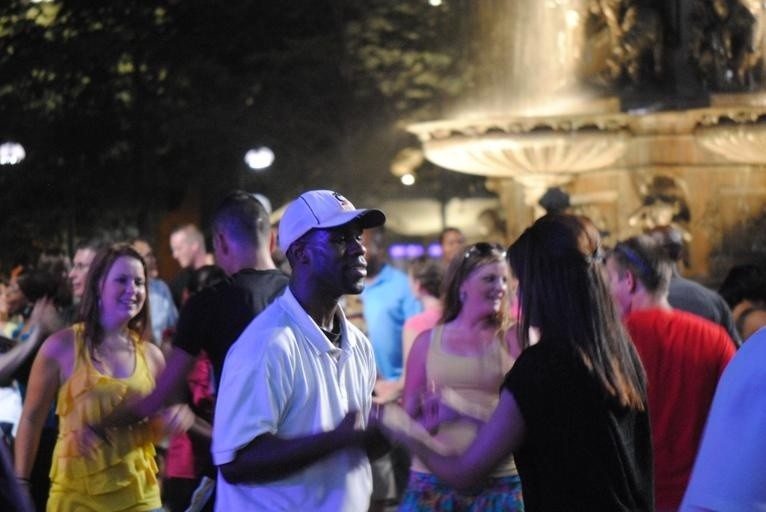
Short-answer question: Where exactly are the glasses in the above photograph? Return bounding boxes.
[461,241,508,265]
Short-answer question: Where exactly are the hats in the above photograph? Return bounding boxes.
[278,189,386,259]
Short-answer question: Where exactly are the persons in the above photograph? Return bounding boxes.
[209,188,398,510]
[0,200,766,511]
[73,189,291,511]
[627,173,694,275]
